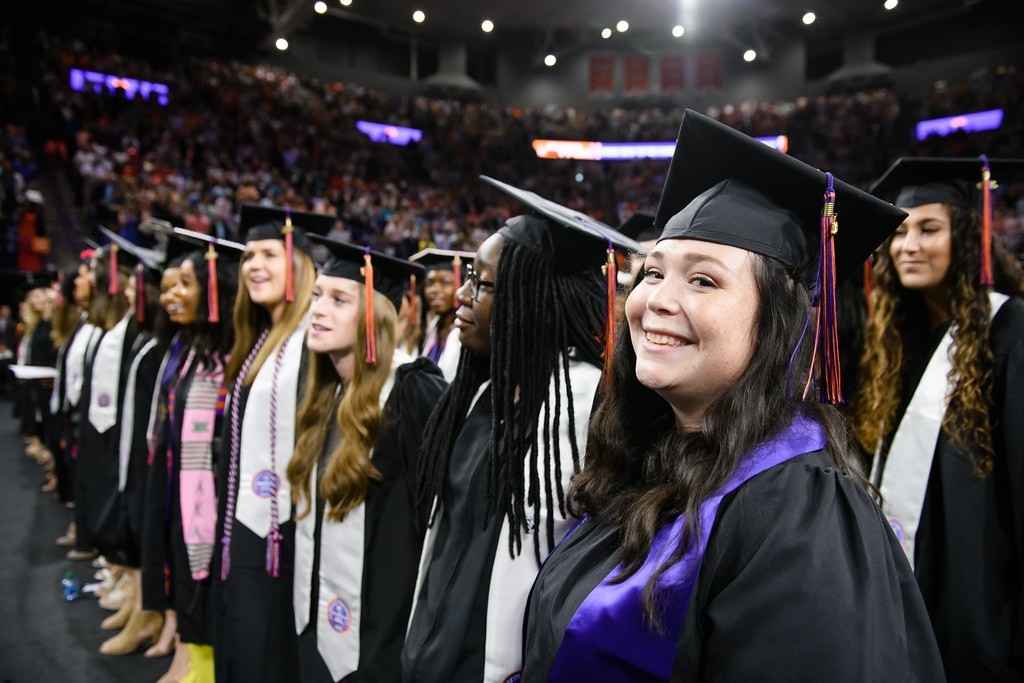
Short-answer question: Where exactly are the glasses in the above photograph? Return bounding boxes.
[465,263,495,300]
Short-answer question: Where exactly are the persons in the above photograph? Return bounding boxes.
[519,110,951,683]
[399,173,646,683]
[0,12,1024,656]
[864,154,1022,683]
[142,226,249,683]
[206,200,341,683]
[283,229,452,682]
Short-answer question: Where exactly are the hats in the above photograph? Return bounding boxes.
[406,247,476,326]
[166,226,247,323]
[654,104,910,406]
[478,168,644,400]
[870,155,1024,285]
[236,203,338,303]
[76,226,162,322]
[302,230,425,367]
